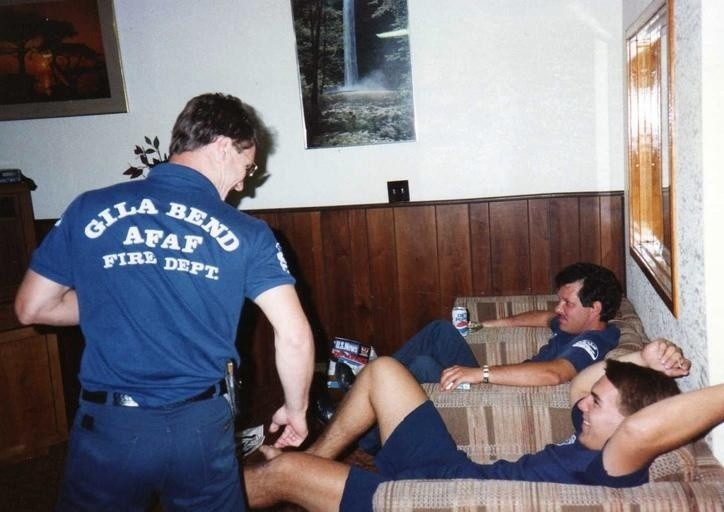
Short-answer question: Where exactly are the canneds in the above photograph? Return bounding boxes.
[451,307,468,338]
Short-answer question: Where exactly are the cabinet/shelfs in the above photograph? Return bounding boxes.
[0,167,68,461]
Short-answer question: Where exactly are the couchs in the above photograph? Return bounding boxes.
[455,295,642,324]
[414,330,648,391]
[373,391,723,512]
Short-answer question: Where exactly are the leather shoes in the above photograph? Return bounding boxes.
[335,361,357,394]
[312,392,338,424]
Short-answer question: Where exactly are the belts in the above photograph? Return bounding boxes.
[76,378,229,412]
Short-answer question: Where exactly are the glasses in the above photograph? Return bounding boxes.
[220,134,258,177]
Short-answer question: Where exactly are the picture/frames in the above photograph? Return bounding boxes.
[622,1,679,319]
[1,0,129,121]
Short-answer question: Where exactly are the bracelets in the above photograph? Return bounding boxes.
[482,364,488,383]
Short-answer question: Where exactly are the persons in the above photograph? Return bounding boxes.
[314,262,623,455]
[241,338,724,512]
[13,92,316,512]
